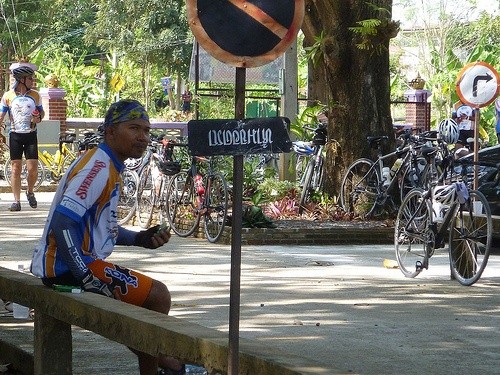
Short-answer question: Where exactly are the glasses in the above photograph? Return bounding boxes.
[26,77,34,80]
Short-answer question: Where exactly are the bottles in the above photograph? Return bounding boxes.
[430,184,443,223]
[390,158,403,176]
[29,109,39,129]
[43,150,54,164]
[413,160,426,181]
[382,165,391,185]
[54,150,60,164]
[194,172,204,195]
[152,169,162,194]
[13,265,29,319]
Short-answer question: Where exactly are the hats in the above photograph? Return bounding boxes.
[104,99,150,127]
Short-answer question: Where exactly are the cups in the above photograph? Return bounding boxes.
[472,201,483,215]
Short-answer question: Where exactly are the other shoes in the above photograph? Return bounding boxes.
[8,203,21,211]
[25,190,37,208]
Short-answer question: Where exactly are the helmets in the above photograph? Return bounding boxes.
[293,141,314,156]
[439,117,460,145]
[156,160,181,176]
[9,63,38,79]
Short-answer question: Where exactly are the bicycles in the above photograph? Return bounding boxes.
[4,126,229,242]
[340,126,500,286]
[299,114,329,213]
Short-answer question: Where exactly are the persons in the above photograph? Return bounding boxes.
[494,100,500,144]
[29,100,187,375]
[447,105,489,158]
[0,66,45,211]
